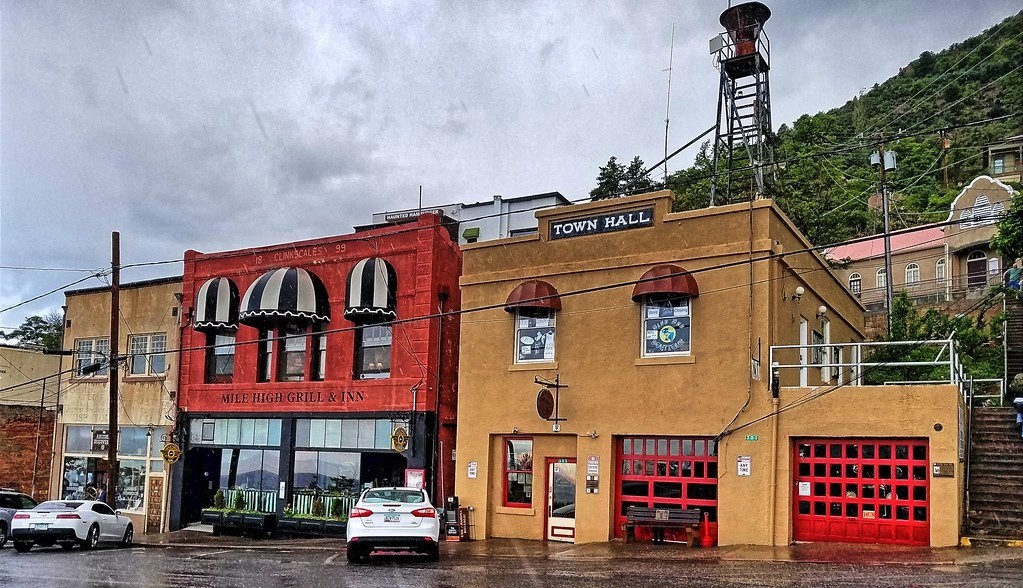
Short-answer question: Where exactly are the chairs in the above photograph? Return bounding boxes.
[406,495,421,502]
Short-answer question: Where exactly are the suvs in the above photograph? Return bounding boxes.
[0,488,39,549]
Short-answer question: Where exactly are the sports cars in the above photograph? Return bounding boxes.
[11,500,134,552]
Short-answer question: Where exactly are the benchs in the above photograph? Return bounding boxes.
[619,504,701,547]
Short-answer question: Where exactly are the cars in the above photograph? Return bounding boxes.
[346,487,440,563]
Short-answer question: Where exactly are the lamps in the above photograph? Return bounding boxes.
[145,427,154,436]
[368,362,375,371]
[816,306,827,318]
[377,362,382,372]
[792,287,804,303]
[174,292,183,305]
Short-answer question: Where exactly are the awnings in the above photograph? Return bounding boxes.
[504,280,562,313]
[193,277,239,332]
[343,257,397,322]
[237,267,331,328]
[631,264,699,302]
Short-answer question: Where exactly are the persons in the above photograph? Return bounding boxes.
[1013,385,1023,439]
[1003,264,1023,299]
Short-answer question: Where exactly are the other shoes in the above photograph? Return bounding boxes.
[1015,422,1022,432]
